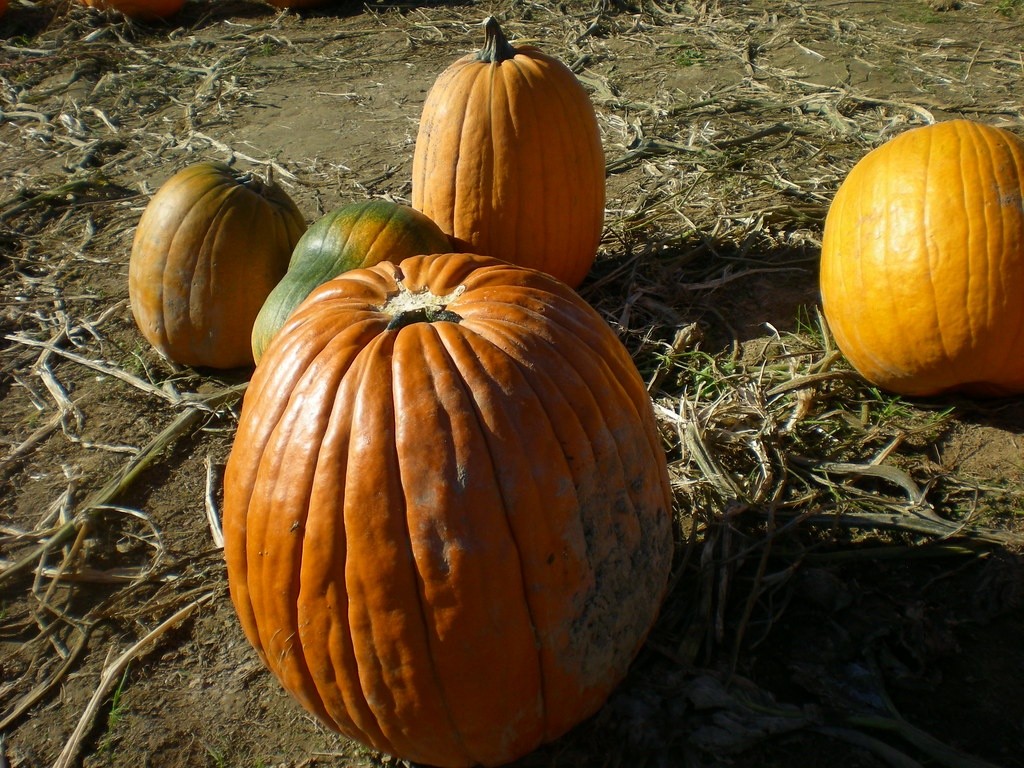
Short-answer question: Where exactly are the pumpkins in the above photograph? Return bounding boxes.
[821,119,1024,398]
[129,16,674,768]
